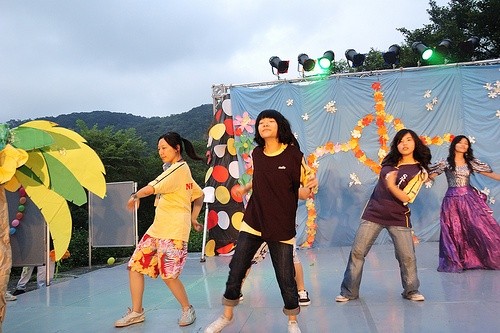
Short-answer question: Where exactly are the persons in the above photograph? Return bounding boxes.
[114,132,205,327]
[5,292,16,301]
[334,128,432,302]
[233,175,312,306]
[10,266,47,295]
[204,109,320,333]
[425,135,500,273]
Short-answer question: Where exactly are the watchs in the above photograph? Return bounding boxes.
[129,192,137,199]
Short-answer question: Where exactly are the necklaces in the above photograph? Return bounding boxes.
[455,158,466,166]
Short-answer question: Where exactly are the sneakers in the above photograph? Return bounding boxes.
[336,296,350,302]
[4,292,17,301]
[287,320,302,333]
[407,293,424,301]
[204,315,236,333]
[12,290,25,296]
[298,289,311,305]
[179,305,197,326]
[114,307,145,326]
[239,290,244,301]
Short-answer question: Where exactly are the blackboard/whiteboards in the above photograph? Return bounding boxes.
[6,190,46,266]
[90,182,135,247]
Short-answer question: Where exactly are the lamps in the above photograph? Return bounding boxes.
[269,37,499,80]
